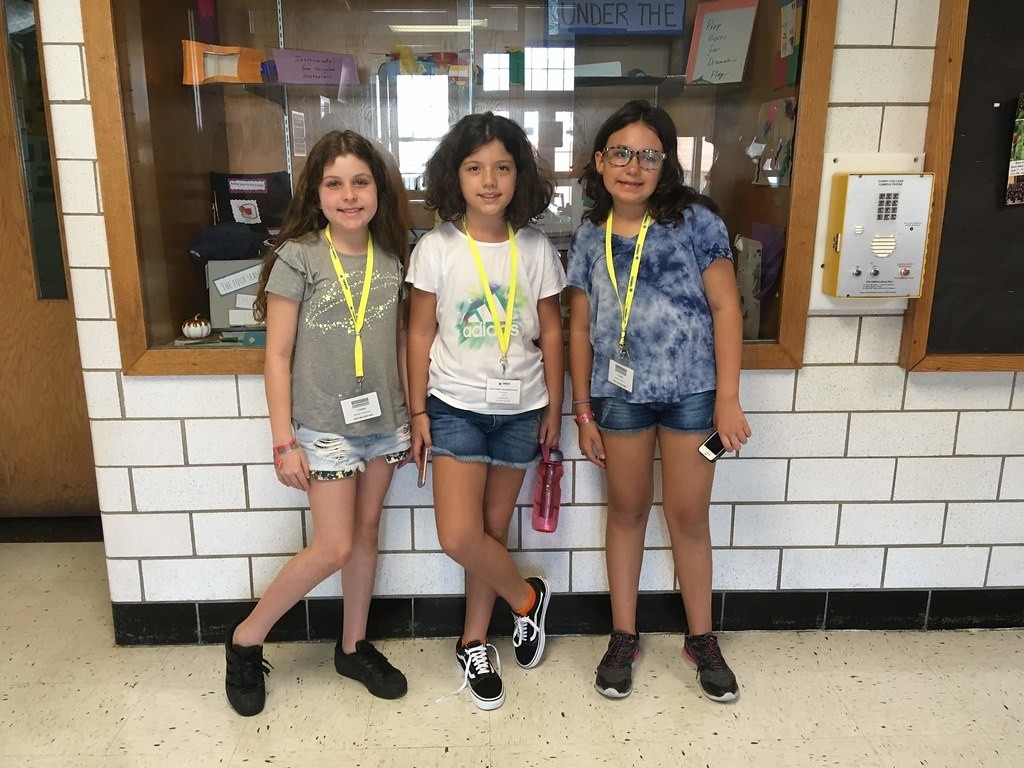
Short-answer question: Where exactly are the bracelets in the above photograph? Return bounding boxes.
[573,399,591,404]
[412,410,427,417]
[574,411,595,426]
[273,439,298,465]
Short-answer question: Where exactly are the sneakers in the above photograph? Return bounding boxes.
[334,633,409,699]
[511,575,551,669]
[681,631,739,702]
[224,616,275,717]
[432,634,507,712]
[595,627,642,698]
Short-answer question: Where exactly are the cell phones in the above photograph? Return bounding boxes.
[699,431,726,463]
[418,443,428,487]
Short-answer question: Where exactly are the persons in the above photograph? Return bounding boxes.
[565,102,752,702]
[404,110,566,711]
[225,129,413,717]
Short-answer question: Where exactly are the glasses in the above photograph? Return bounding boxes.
[602,145,667,170]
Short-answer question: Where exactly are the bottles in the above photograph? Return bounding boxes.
[531,441,564,533]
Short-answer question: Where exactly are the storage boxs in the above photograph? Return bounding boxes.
[736,237,762,339]
[207,260,267,332]
